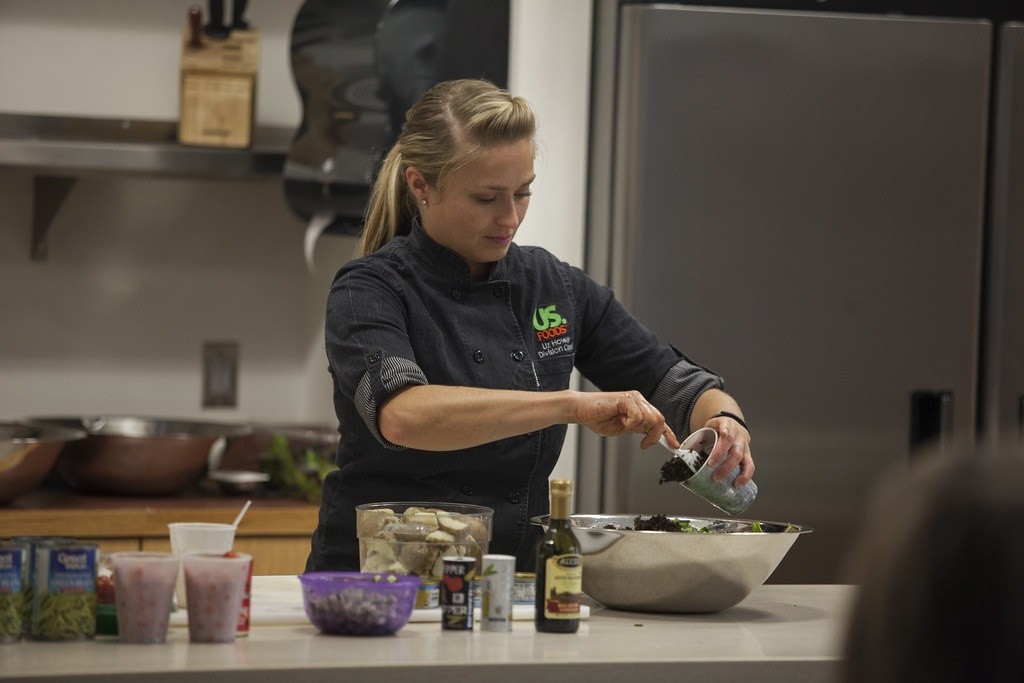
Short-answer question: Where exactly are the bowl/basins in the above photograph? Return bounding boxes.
[0,421,88,503]
[531,515,813,613]
[36,416,256,493]
[297,572,422,637]
[354,501,494,580]
[205,469,271,496]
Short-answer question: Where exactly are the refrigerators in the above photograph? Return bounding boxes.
[578,1,1024,583]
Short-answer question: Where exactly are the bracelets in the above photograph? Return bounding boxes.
[710,411,751,432]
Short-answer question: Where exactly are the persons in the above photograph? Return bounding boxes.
[838,444,1024,683]
[303,80,756,573]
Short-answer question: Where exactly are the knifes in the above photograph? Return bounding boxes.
[181,0,255,47]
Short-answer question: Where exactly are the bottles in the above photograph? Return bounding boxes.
[535,480,582,634]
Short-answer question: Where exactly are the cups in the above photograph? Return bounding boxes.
[168,522,237,609]
[673,427,759,516]
[182,554,253,645]
[109,551,181,644]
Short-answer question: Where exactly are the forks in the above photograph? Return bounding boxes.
[659,434,703,473]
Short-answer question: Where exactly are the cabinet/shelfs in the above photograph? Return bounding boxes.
[0,498,321,579]
